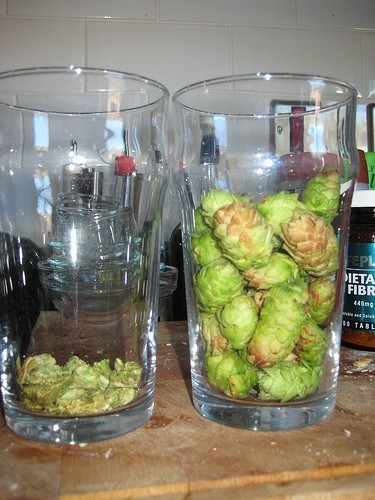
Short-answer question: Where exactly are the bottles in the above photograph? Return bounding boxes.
[112,156,139,241]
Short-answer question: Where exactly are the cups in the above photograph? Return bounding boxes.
[168,74,355,430]
[0,66,173,445]
